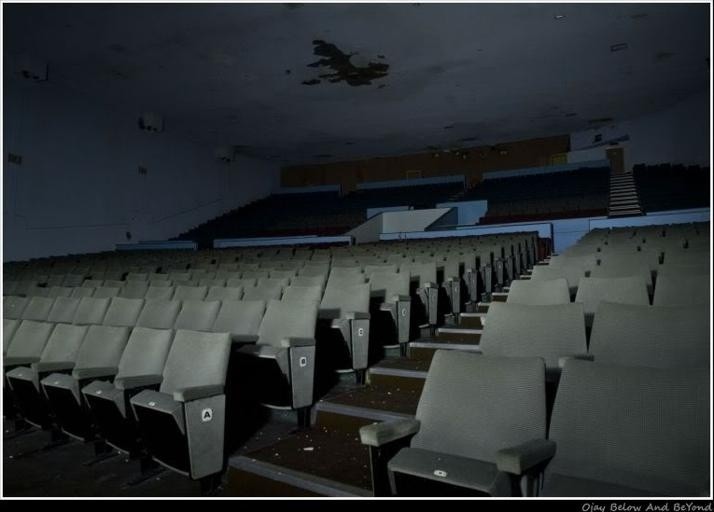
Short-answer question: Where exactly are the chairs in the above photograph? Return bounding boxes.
[358,220,710,496]
[3,229,545,480]
